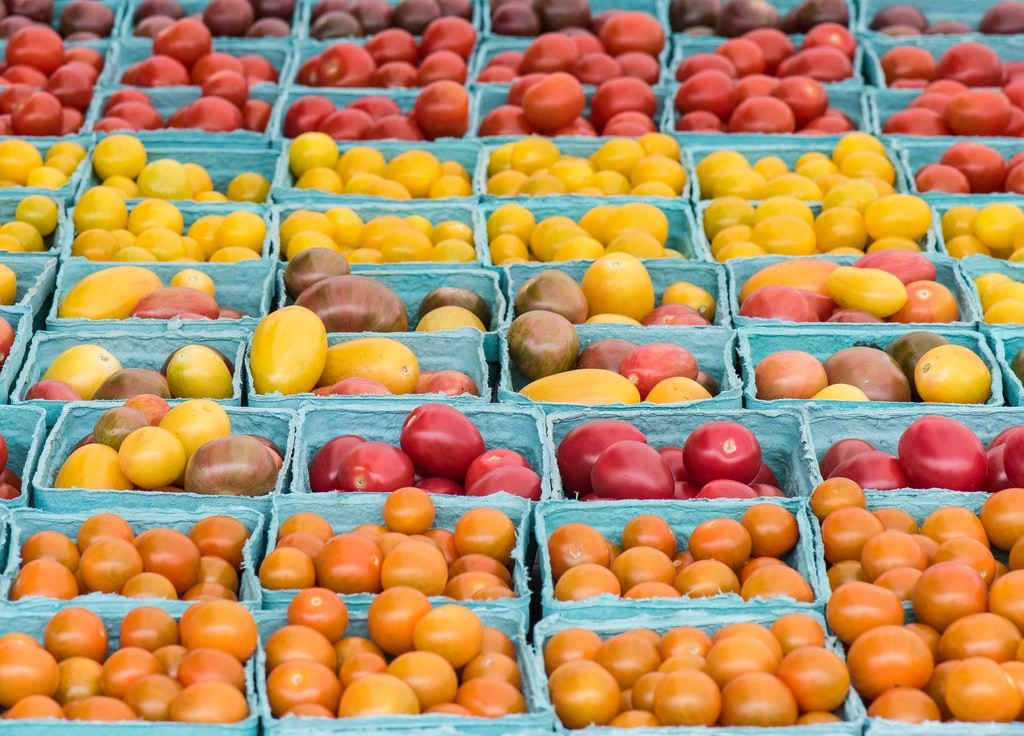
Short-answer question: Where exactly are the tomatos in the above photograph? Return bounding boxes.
[0,0,1024,729]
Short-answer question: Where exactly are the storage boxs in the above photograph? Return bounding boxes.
[0,0,1024,736]
